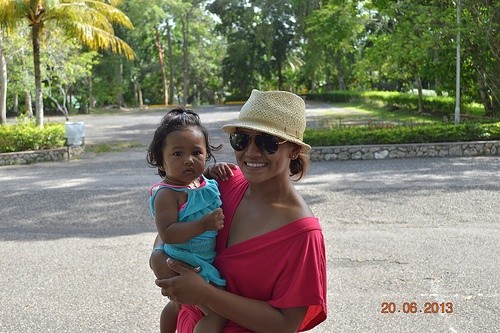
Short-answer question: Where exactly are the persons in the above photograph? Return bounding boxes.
[149,88,327,333]
[146,107,238,333]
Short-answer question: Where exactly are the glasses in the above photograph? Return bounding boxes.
[229,130,288,154]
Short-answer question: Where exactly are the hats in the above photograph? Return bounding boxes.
[222,89,312,153]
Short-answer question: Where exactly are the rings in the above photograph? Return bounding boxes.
[169,296,172,301]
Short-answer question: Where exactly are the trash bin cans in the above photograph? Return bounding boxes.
[65,120,85,146]
